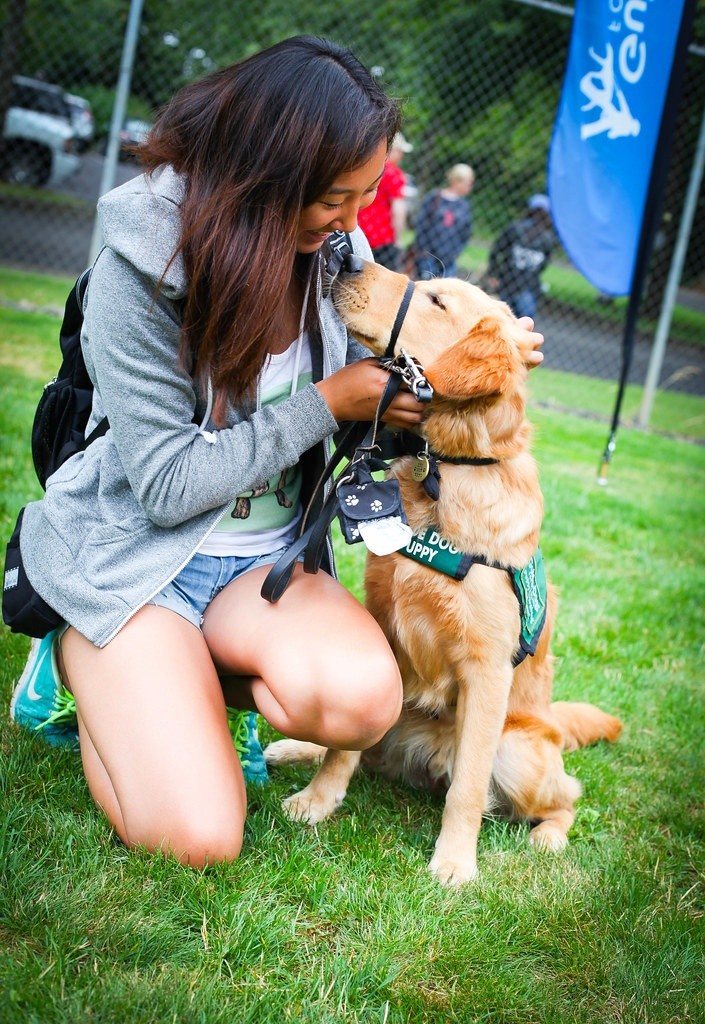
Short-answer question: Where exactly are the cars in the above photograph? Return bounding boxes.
[118,119,155,163]
[3,74,99,194]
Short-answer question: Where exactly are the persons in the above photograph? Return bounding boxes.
[402,163,475,282]
[8,33,542,873]
[484,192,561,319]
[356,129,415,273]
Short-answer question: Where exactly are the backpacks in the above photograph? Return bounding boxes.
[30,224,354,494]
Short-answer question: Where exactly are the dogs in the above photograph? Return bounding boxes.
[260,254,626,886]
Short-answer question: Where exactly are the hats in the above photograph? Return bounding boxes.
[390,132,414,153]
[527,193,550,213]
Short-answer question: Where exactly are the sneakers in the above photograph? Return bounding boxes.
[10,630,80,756]
[225,706,271,794]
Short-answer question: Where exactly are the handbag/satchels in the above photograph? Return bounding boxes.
[0,506,65,640]
